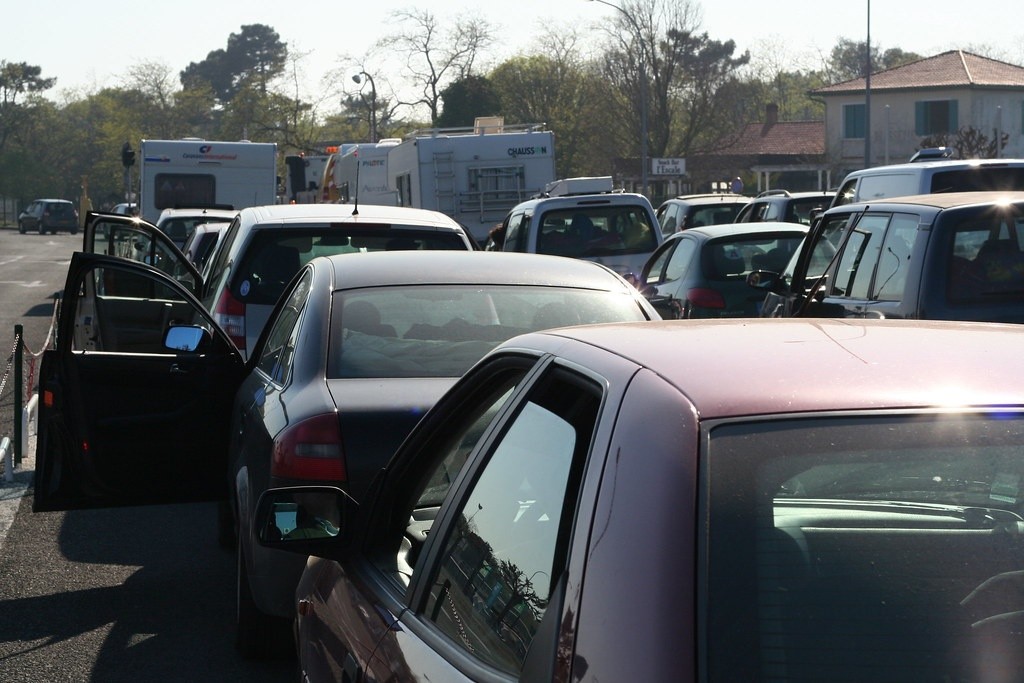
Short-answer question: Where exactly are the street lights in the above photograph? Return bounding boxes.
[352,71,377,144]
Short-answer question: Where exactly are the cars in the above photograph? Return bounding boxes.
[103,202,137,240]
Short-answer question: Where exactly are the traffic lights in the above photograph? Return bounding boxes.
[122,142,135,168]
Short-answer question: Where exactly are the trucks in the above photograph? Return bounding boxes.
[135,136,280,265]
[284,114,554,248]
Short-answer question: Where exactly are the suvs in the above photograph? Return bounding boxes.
[18,198,79,235]
[32,148,1024,682]
